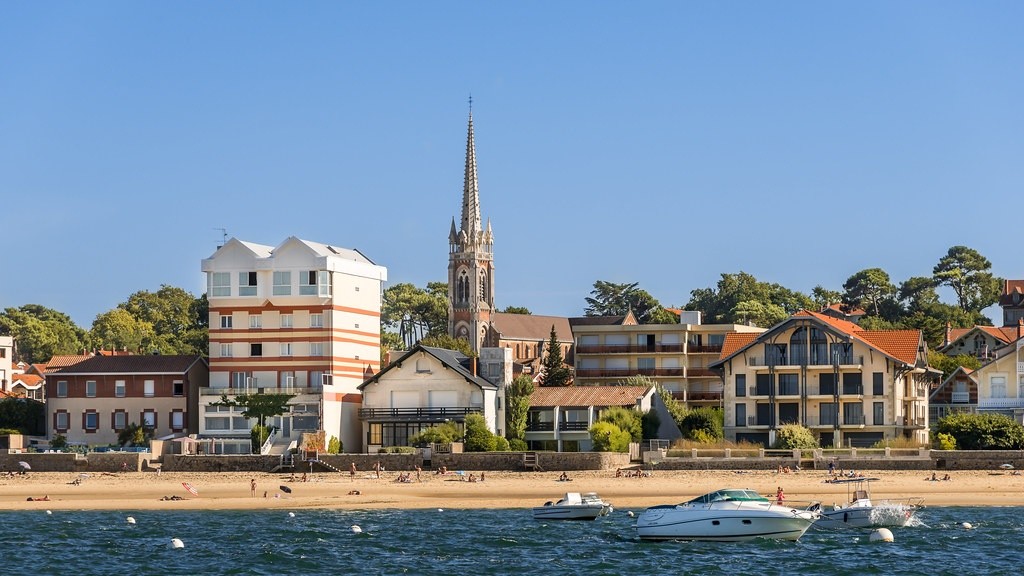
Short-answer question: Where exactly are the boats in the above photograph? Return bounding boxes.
[999,464,1013,470]
[814,489,925,528]
[632,487,821,543]
[532,492,603,521]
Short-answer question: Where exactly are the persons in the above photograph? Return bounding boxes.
[468,472,485,482]
[162,495,182,501]
[375,462,380,478]
[301,473,306,482]
[783,466,790,473]
[25,478,81,501]
[250,479,257,498]
[774,486,785,506]
[615,467,651,477]
[931,473,949,481]
[414,465,423,482]
[6,468,26,476]
[397,472,413,483]
[560,472,569,481]
[350,463,356,482]
[290,473,295,482]
[827,463,859,477]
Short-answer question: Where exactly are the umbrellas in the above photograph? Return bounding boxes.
[181,482,198,497]
[19,461,31,469]
[280,485,291,493]
[171,437,200,452]
